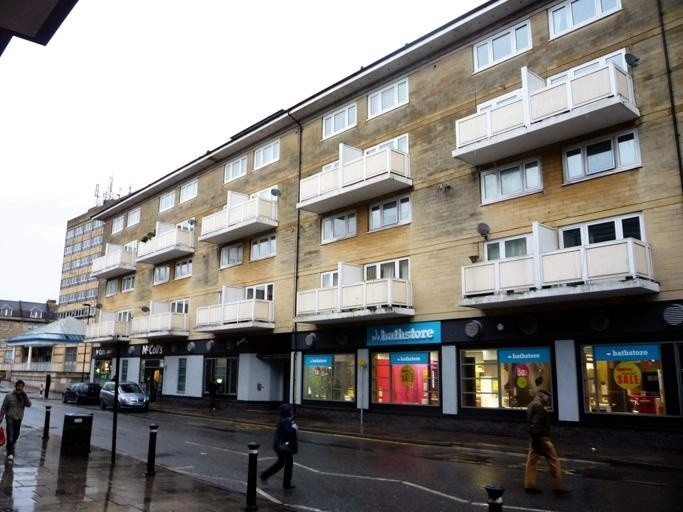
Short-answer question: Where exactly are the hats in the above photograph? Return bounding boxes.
[538,389,552,396]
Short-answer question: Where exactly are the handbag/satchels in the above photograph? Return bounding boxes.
[279,438,298,453]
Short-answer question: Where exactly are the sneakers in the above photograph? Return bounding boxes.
[8,454,13,460]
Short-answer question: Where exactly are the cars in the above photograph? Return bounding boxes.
[60,382,101,405]
[98,378,150,412]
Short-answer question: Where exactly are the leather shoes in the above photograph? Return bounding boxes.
[283,485,296,489]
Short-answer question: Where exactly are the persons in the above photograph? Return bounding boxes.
[40,384,43,397]
[0,379,31,460]
[524,389,573,495]
[258,404,298,491]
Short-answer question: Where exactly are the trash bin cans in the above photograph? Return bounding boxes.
[60,412,93,456]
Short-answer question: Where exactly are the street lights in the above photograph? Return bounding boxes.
[78,302,90,381]
